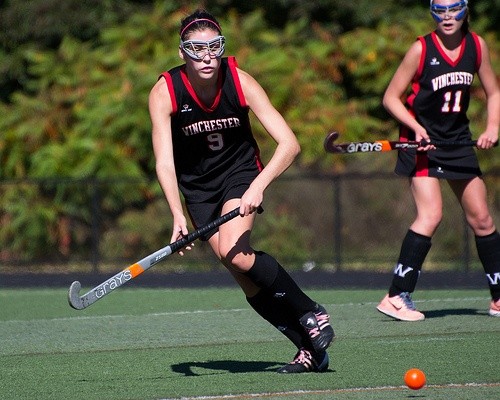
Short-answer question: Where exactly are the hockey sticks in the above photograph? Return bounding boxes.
[324,131,499,154]
[68,206,240,310]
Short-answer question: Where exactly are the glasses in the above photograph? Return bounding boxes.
[180,36,226,61]
[430,2,468,23]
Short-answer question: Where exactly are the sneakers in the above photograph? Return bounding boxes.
[376,291,425,321]
[276,345,330,374]
[489,299,500,317]
[299,303,335,352]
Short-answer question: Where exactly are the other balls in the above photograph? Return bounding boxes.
[404,369,426,390]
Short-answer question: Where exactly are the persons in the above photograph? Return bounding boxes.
[149,10,335,373]
[375,0,500,321]
